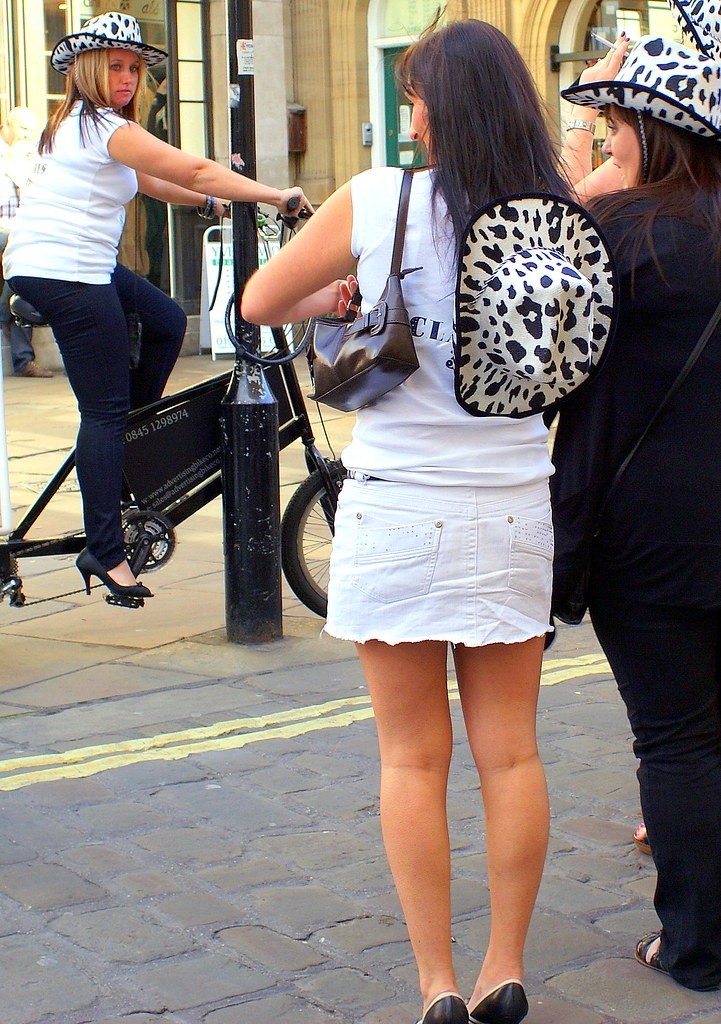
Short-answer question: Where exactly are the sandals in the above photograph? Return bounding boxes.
[633,830,652,856]
[634,931,671,976]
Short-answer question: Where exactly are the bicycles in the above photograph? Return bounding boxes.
[0,196,349,618]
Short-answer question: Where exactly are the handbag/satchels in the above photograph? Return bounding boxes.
[308,169,421,413]
[553,519,595,626]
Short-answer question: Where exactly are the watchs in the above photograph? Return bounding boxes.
[566,120,597,136]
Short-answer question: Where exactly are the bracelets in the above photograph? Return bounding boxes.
[196,194,217,220]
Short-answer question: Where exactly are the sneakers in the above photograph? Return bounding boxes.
[14,362,54,377]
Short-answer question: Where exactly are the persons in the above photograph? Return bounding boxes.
[241,20,612,1024]
[0,10,313,596]
[546,32,721,991]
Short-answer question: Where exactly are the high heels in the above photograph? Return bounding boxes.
[75,545,154,601]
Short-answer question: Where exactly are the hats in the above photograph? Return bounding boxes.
[453,193,617,420]
[51,13,168,76]
[560,34,721,139]
[669,0,721,59]
